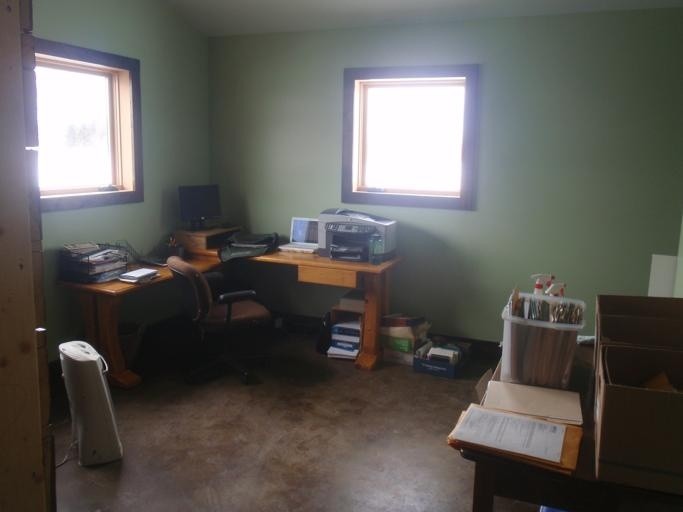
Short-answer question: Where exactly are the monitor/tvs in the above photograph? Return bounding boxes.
[179,184,222,232]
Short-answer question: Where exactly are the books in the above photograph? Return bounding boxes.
[64,240,161,285]
[326,320,361,359]
[453,284,584,463]
[380,311,467,378]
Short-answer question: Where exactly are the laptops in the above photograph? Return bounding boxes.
[278,217,318,254]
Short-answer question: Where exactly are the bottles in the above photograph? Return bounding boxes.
[368,234,384,265]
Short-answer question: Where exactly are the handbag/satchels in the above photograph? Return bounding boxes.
[216,231,277,263]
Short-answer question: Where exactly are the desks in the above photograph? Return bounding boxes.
[54,236,406,390]
[461,335,652,512]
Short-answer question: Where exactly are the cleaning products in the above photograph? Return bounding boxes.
[530,273,555,297]
[545,281,567,297]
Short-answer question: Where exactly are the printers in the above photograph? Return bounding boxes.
[318,208,397,265]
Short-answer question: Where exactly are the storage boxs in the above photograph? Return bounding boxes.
[593,295,683,497]
[498,291,585,391]
